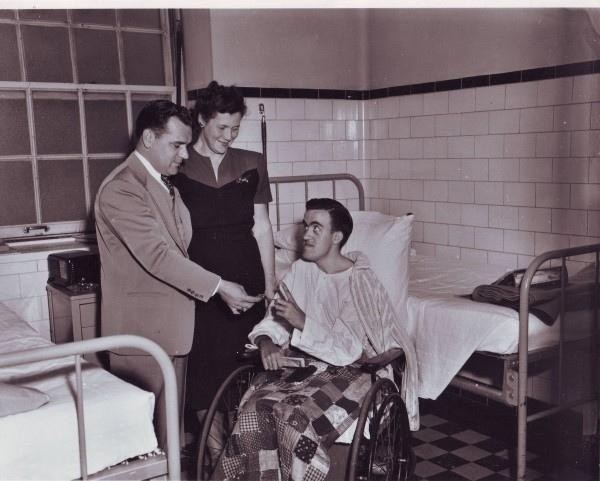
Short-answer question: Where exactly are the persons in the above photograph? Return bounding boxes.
[95,100,266,481]
[248,198,419,444]
[161,81,276,481]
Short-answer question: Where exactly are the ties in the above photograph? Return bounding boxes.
[161,175,174,197]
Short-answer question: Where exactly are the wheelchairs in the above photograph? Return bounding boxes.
[197,341,412,481]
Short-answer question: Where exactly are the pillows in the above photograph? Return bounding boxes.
[342,210,412,335]
[0,380,48,419]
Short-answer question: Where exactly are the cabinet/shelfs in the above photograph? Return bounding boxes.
[47,286,102,369]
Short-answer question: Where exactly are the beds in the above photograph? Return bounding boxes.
[255,173,600,480]
[0,290,180,481]
